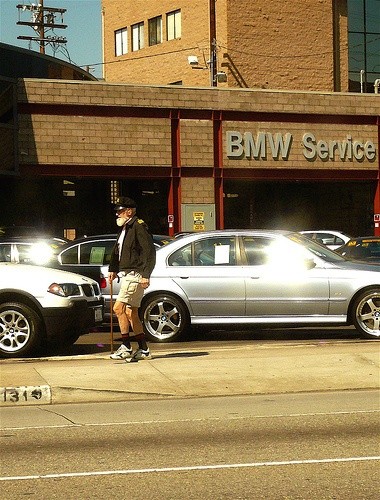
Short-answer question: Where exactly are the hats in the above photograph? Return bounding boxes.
[113,198,136,210]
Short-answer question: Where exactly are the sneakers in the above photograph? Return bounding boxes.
[126,347,151,360]
[110,344,133,360]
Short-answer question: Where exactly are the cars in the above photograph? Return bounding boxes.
[336,238,380,264]
[0,264,105,359]
[49,234,215,287]
[302,230,351,251]
[0,236,73,269]
[101,229,380,344]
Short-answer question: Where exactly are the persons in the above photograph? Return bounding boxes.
[108,196,156,363]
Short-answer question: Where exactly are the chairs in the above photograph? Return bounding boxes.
[197,250,215,265]
